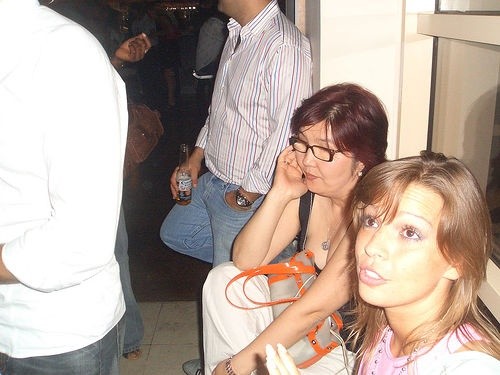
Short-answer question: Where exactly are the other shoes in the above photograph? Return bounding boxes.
[183,359,206,375]
[122,347,141,360]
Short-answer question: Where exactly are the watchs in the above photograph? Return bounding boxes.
[236,189,252,206]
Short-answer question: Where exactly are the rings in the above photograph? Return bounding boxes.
[145,50,148,53]
[284,161,290,164]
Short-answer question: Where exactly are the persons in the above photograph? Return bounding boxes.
[159,0,312,375]
[0,1,128,375]
[38,1,230,359]
[268,150,500,375]
[201,81,389,375]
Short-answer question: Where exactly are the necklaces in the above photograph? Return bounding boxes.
[322,200,343,250]
[372,329,427,375]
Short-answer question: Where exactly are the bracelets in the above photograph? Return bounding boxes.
[225,357,235,375]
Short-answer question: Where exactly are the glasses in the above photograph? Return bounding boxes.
[289,136,349,162]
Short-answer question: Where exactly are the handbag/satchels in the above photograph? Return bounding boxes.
[124,103,165,180]
[226,250,344,369]
